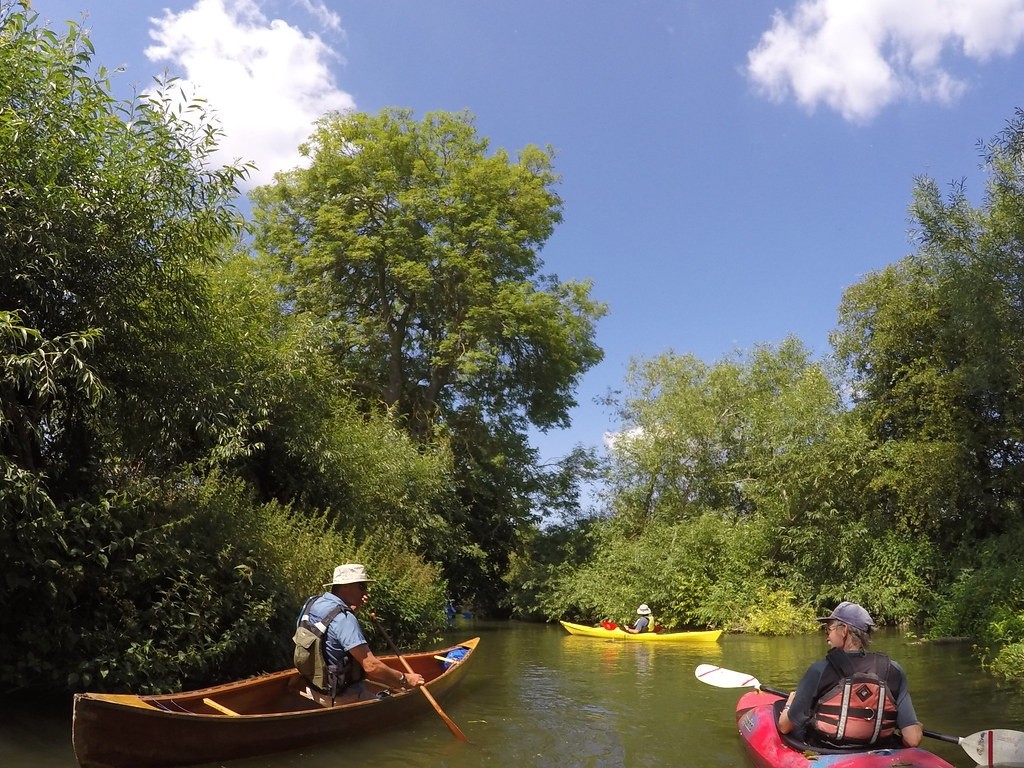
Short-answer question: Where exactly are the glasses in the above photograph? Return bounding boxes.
[820,625,843,631]
[356,582,367,591]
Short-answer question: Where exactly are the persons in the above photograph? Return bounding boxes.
[623,604,655,634]
[778,601,923,750]
[296,563,425,707]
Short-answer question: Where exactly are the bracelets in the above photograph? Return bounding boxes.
[784,705,790,709]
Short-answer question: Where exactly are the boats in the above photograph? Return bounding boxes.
[72,636,484,768]
[737,688,957,768]
[559,620,724,642]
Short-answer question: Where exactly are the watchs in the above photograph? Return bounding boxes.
[399,672,407,686]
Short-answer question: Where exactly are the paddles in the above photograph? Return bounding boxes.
[600,621,662,632]
[369,612,468,742]
[693,662,1024,768]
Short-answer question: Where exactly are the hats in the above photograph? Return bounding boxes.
[815,601,876,634]
[637,604,651,615]
[322,564,376,587]
